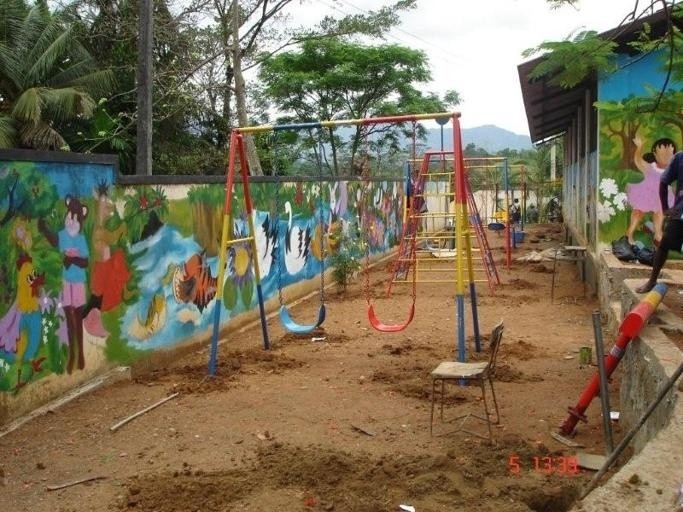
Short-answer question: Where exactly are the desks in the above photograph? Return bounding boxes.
[553,246,589,299]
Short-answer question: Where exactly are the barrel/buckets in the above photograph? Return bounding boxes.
[514,231,524,242]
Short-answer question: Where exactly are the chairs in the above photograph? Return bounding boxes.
[427,321,505,444]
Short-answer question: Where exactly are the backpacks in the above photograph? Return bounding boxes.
[513,204,521,218]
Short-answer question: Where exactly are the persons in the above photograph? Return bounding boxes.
[635,149,682,294]
[511,197,521,230]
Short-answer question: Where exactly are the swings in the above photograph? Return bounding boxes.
[362,119,416,332]
[272,127,325,334]
